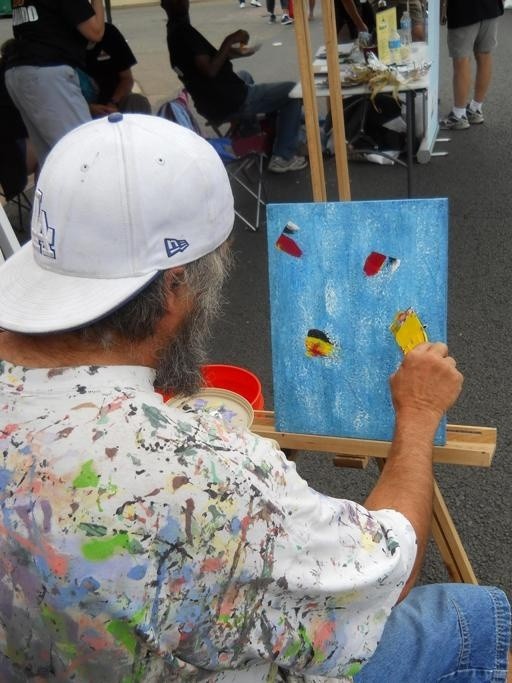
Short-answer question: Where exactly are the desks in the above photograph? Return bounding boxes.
[287,41,432,199]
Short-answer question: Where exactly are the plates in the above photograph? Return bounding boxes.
[165,385,257,436]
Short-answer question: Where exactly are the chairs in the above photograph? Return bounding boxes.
[161,73,277,232]
[0,179,35,227]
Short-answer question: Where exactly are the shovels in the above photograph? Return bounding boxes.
[388,306,428,355]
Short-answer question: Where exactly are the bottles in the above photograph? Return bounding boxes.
[388,26,402,64]
[400,10,412,48]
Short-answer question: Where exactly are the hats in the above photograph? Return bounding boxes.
[0,112,235,335]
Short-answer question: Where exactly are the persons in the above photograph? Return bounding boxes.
[1,1,505,202]
[0,107,512,681]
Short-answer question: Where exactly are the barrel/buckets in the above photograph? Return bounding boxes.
[155,364,265,418]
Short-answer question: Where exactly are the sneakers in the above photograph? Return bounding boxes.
[438,104,484,129]
[268,156,308,172]
[240,0,294,25]
